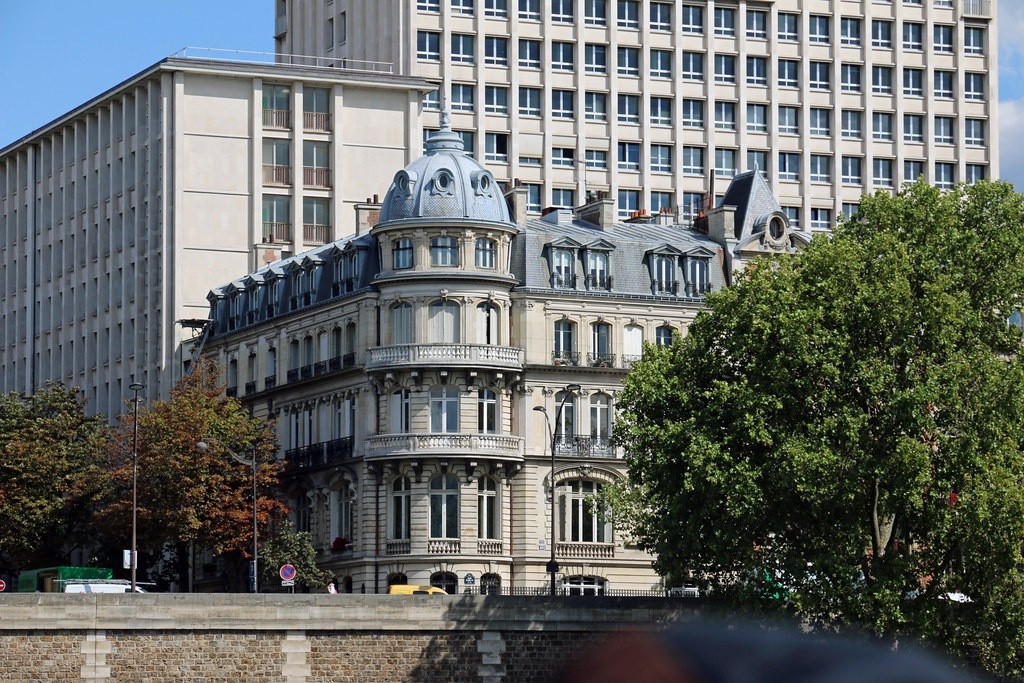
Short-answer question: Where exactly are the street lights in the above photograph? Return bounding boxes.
[128,381,145,594]
[532,383,582,597]
[195,436,257,595]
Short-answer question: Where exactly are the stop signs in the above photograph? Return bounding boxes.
[279,563,296,580]
[0,580,5,591]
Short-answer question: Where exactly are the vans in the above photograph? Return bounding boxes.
[63,578,148,595]
[388,584,450,596]
